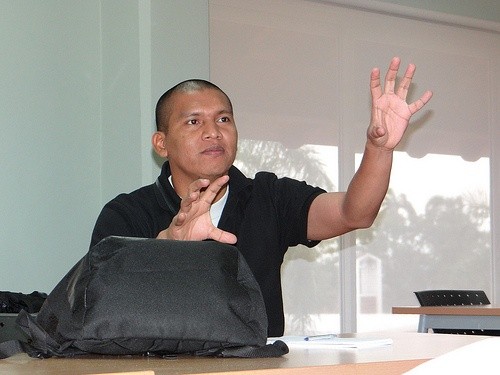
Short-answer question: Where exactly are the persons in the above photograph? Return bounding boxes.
[86,54,435,339]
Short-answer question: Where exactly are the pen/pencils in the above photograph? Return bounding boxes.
[303,334,338,341]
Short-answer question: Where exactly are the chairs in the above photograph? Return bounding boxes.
[414,290,500,337]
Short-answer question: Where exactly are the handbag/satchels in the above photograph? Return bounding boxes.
[14,235,290,359]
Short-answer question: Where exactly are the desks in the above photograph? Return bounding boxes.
[392,306,500,334]
